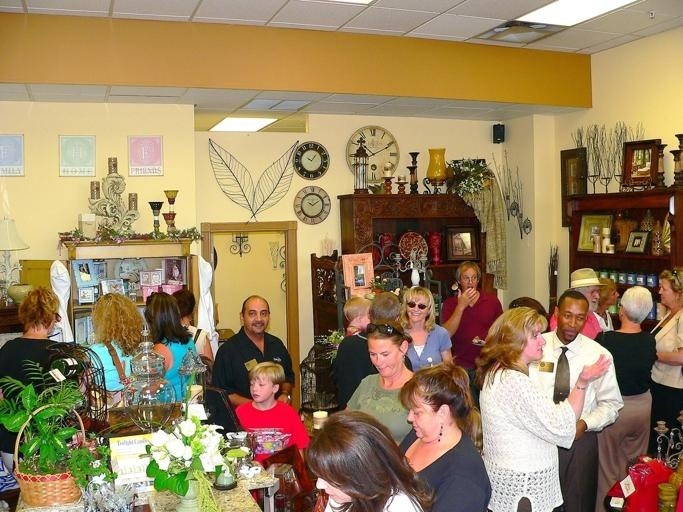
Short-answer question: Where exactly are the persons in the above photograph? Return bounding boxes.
[144,292,196,402]
[85,293,145,409]
[0,284,84,512]
[172,289,213,364]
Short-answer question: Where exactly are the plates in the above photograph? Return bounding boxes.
[473,343,487,348]
[397,230,428,261]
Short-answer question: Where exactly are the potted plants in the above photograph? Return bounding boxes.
[0,355,95,505]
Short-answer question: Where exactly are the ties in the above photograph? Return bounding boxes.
[554,347,571,404]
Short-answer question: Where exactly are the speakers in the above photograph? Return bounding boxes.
[493,124,505,143]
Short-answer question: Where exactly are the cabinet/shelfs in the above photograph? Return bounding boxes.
[336,193,497,325]
[566,187,682,334]
[62,238,199,348]
[0,303,30,333]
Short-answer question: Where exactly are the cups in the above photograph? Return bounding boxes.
[607,244,615,253]
[128,192,138,211]
[593,235,601,253]
[90,180,100,200]
[107,157,116,174]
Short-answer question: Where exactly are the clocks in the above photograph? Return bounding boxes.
[345,126,400,182]
[292,141,329,180]
[293,185,330,224]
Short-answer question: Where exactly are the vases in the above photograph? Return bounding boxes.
[7,284,32,305]
[424,148,452,195]
[371,288,382,295]
[171,476,202,512]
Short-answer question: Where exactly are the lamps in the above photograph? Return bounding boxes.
[0,215,32,306]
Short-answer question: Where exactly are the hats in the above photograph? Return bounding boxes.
[565,267,608,291]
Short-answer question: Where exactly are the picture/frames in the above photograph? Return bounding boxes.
[621,138,661,187]
[559,148,587,228]
[55,134,97,179]
[0,134,26,178]
[126,136,163,177]
[70,258,186,306]
[622,230,650,253]
[441,225,480,265]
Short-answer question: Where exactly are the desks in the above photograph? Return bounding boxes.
[15,457,273,512]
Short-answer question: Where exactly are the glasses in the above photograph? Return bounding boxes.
[54,313,62,322]
[366,323,403,338]
[407,301,429,309]
[671,268,682,287]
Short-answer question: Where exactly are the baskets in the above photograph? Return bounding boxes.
[12,403,91,506]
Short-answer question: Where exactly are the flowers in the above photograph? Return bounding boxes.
[366,274,388,289]
[314,328,345,364]
[446,160,494,198]
[95,369,223,511]
[57,224,203,255]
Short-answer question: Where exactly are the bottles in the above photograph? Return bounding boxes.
[178,347,207,423]
[122,323,175,434]
[602,227,610,253]
[658,483,677,512]
